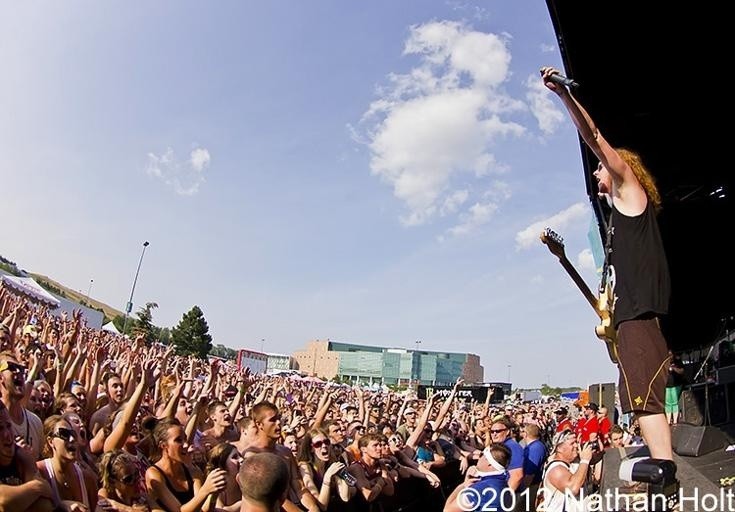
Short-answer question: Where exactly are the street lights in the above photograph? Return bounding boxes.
[507,363,512,384]
[262,337,266,353]
[415,340,422,351]
[124,241,150,337]
[86,278,94,305]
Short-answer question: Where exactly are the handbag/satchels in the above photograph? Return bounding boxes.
[535,481,545,512]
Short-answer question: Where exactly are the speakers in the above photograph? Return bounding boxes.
[671,425,725,457]
[704,382,729,428]
[679,383,707,426]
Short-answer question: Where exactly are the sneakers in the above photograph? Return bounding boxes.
[619,481,679,512]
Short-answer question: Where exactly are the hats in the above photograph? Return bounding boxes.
[550,432,576,455]
[554,407,567,414]
[584,403,598,411]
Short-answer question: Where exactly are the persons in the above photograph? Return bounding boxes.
[539,64,683,511]
[663,348,687,427]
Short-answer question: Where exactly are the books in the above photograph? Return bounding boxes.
[0,267,647,511]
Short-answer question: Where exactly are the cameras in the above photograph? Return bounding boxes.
[582,441,599,452]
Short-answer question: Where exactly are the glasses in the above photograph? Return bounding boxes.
[406,412,416,415]
[556,429,571,442]
[311,439,330,448]
[0,360,25,373]
[109,468,139,485]
[423,428,433,434]
[599,162,603,171]
[390,437,400,442]
[490,428,507,433]
[50,428,77,440]
[68,380,82,386]
[374,410,380,412]
[479,453,483,458]
[355,426,366,430]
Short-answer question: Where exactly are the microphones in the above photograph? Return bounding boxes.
[540,66,579,90]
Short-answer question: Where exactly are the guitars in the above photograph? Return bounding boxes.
[542,227,618,365]
